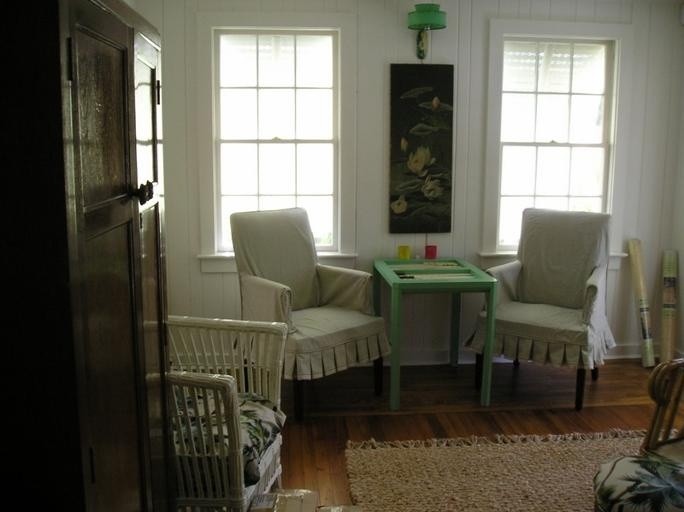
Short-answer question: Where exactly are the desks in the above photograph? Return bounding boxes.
[373,255,498,409]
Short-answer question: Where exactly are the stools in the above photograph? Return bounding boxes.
[592,454,683,512]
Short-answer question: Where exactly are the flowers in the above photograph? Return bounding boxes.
[391,136,451,233]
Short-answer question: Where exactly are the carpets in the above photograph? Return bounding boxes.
[338,427,684,512]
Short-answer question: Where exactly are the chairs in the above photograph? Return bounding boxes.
[229,207,391,419]
[156,306,288,512]
[637,359,684,475]
[467,207,616,410]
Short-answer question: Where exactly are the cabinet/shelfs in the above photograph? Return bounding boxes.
[0,0,175,511]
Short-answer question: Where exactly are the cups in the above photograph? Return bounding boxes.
[424,244,437,259]
[397,245,411,259]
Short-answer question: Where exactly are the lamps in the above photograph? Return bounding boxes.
[409,4,446,60]
